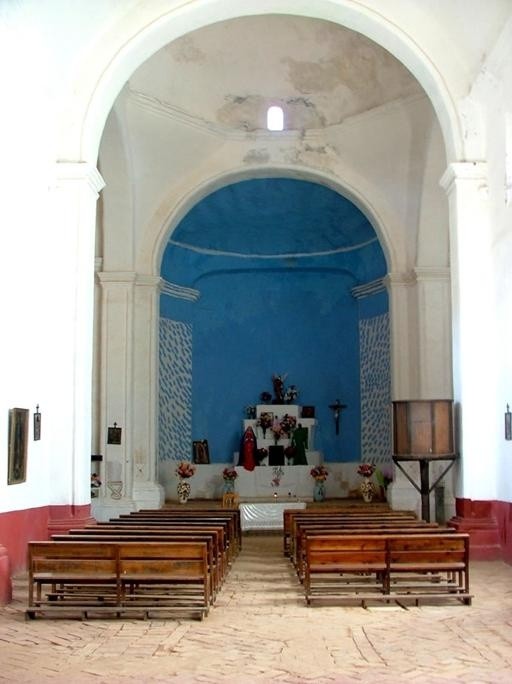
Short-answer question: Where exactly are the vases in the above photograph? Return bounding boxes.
[312,478,327,501]
[222,479,235,495]
[360,474,375,502]
[177,477,191,506]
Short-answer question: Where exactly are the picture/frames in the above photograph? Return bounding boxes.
[8,407,29,486]
[504,412,512,440]
[34,413,42,440]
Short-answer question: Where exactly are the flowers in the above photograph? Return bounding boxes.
[310,464,329,485]
[222,468,240,483]
[354,461,377,484]
[173,460,196,483]
[243,373,311,465]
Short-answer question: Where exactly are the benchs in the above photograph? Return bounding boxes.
[27,504,243,621]
[281,507,475,610]
